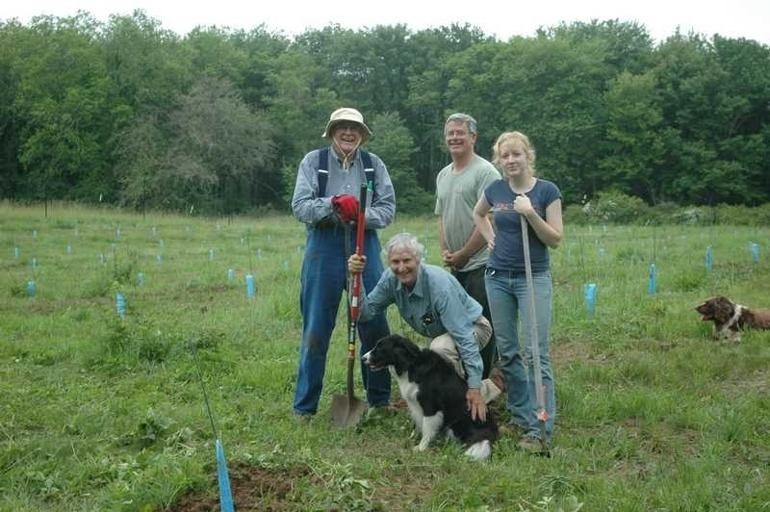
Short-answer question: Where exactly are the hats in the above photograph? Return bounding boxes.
[320,105,373,141]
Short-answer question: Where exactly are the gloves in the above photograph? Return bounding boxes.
[332,193,361,218]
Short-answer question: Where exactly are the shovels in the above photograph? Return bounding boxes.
[332,184,367,428]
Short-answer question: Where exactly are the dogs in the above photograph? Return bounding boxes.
[693,294,770,346]
[361,332,499,464]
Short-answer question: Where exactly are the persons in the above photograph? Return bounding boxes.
[431,112,505,381]
[345,231,508,425]
[291,106,394,417]
[471,131,564,453]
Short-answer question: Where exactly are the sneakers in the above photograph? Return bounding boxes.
[490,367,509,393]
[366,406,398,419]
[498,420,550,456]
